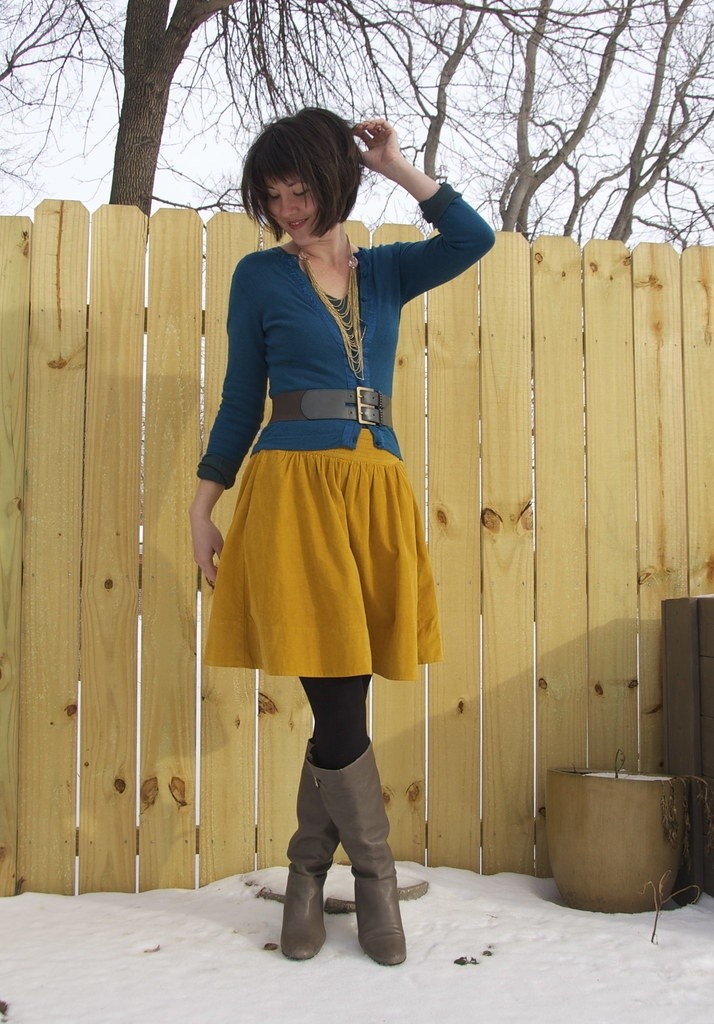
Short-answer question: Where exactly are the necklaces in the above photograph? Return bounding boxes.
[297,233,366,380]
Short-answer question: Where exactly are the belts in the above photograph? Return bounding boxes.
[271,386,393,430]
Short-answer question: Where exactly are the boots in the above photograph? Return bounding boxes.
[280,735,341,962]
[306,736,408,967]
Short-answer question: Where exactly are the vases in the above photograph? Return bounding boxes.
[544,768,687,914]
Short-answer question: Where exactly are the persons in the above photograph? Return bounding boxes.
[187,107,497,966]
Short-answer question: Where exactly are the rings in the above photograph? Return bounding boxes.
[378,127,382,132]
